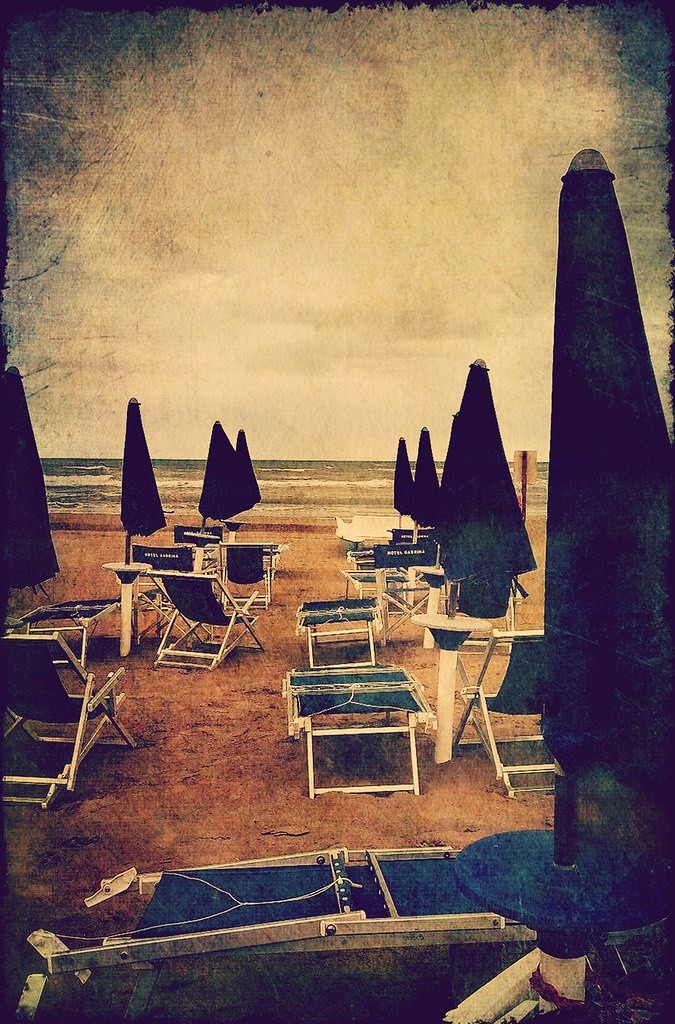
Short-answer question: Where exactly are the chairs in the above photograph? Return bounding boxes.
[131,541,204,621]
[461,628,566,796]
[336,515,413,544]
[283,663,437,798]
[26,846,654,988]
[10,598,122,669]
[292,596,390,670]
[0,633,138,810]
[338,569,424,604]
[345,550,375,567]
[141,571,264,671]
[218,540,286,610]
[174,525,224,566]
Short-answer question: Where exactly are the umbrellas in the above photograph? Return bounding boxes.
[394,437,414,528]
[235,429,262,505]
[412,427,440,541]
[436,358,537,619]
[121,398,167,567]
[0,367,60,590]
[541,149,675,868]
[198,421,260,532]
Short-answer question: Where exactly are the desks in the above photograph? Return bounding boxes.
[456,829,669,1024]
[184,531,219,573]
[219,520,248,540]
[411,614,491,765]
[418,567,446,650]
[103,562,152,656]
